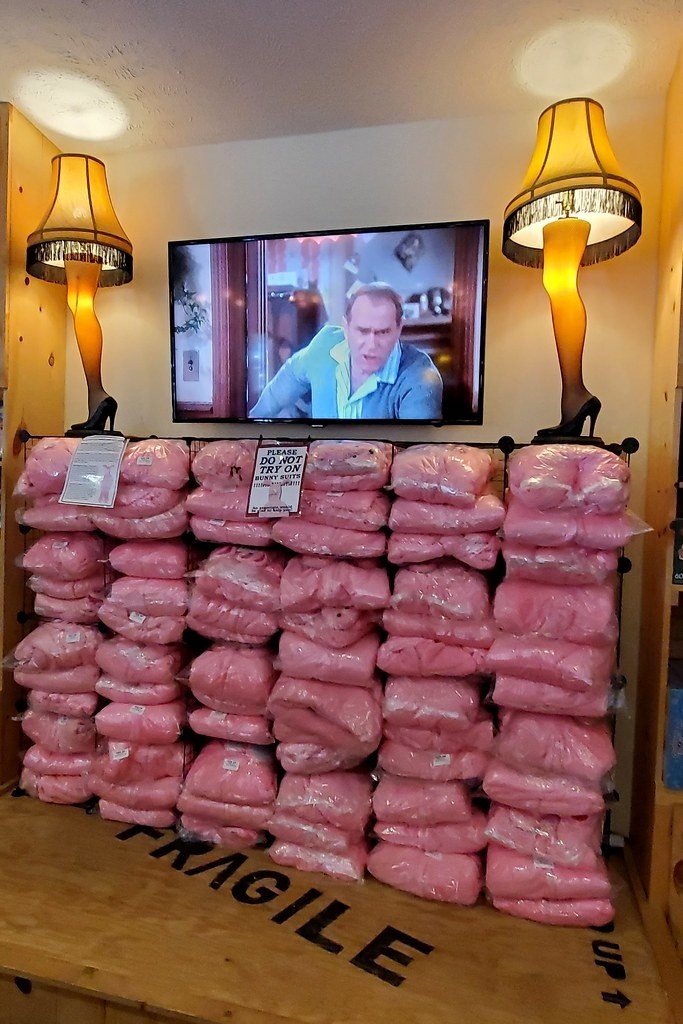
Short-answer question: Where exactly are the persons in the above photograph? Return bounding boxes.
[250,282,443,420]
[63,253,118,430]
[537,217,602,437]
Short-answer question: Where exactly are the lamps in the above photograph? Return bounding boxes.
[23,154,133,434]
[501,97,646,442]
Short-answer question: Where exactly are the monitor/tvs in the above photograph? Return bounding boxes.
[169,218,490,425]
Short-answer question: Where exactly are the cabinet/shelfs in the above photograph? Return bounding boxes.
[629,49,683,920]
